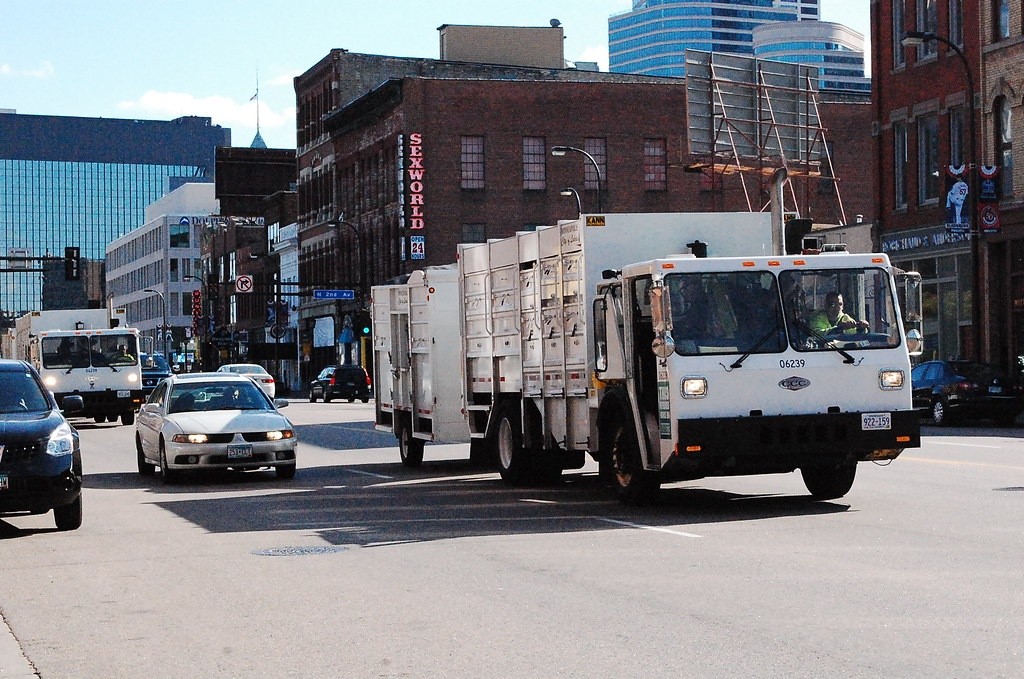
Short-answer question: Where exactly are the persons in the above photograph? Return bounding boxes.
[944,173,969,224]
[110,345,135,362]
[808,291,870,349]
[225,386,238,403]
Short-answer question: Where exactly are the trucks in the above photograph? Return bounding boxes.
[1,291,143,427]
[370,166,926,504]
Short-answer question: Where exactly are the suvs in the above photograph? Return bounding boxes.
[0,358,85,530]
[139,354,180,410]
[308,365,372,404]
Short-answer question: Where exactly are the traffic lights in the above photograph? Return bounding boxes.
[361,316,372,336]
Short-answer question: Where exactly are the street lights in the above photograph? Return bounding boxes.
[327,219,367,315]
[144,289,167,362]
[183,275,208,374]
[249,252,282,373]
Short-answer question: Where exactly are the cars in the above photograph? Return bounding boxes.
[135,372,298,485]
[911,359,1021,427]
[216,364,275,402]
